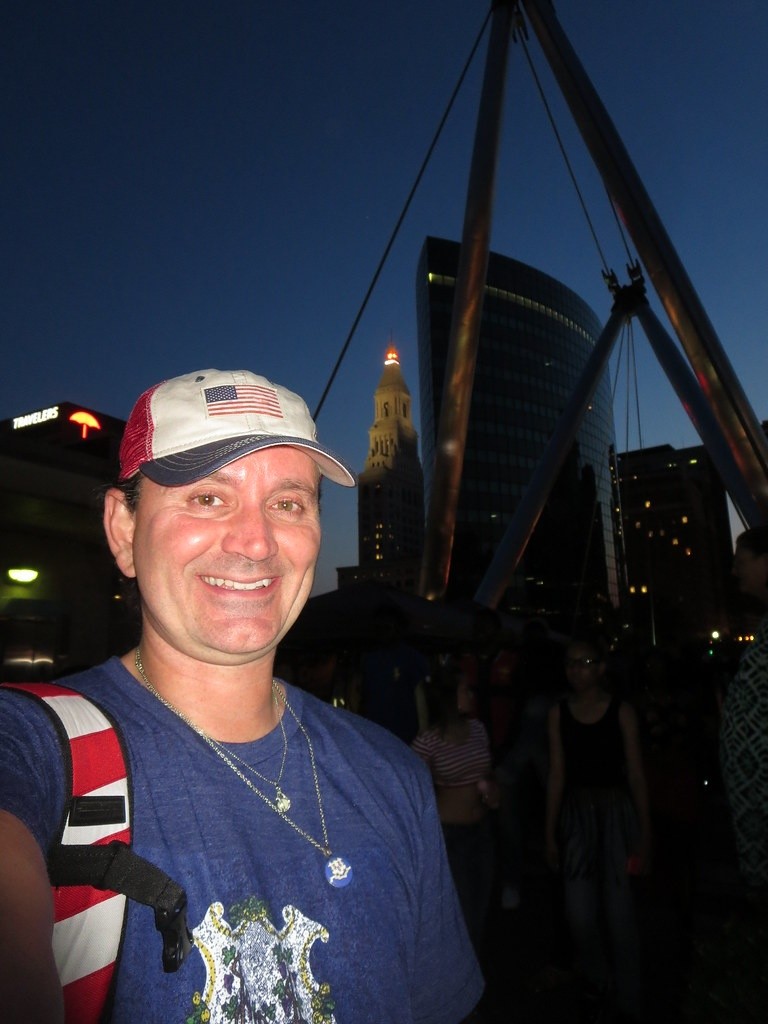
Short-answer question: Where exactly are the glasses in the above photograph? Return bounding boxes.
[565,656,597,667]
[731,557,759,568]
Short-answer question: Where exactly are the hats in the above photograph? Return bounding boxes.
[112,366,358,491]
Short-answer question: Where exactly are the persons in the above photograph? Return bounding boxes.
[716,653,736,708]
[409,664,501,890]
[544,635,652,1020]
[444,608,515,768]
[716,526,768,934]
[0,365,489,1024]
[510,618,567,737]
[349,603,429,747]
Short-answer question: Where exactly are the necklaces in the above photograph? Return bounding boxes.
[135,646,353,885]
[136,654,293,813]
